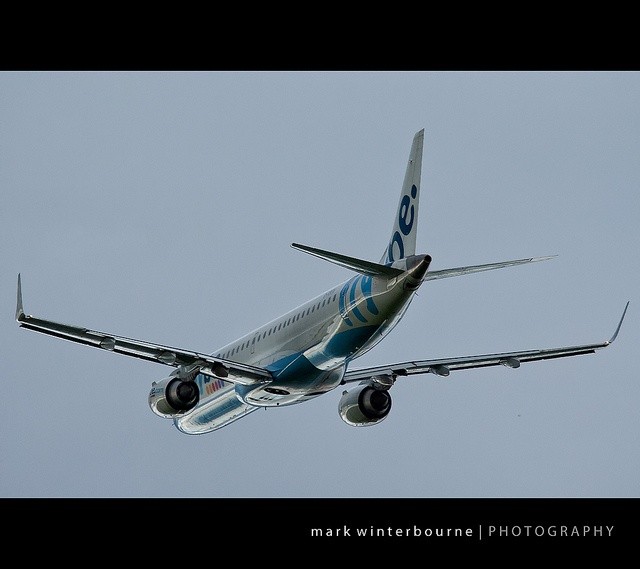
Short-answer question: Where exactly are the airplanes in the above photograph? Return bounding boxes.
[15,128,631,435]
